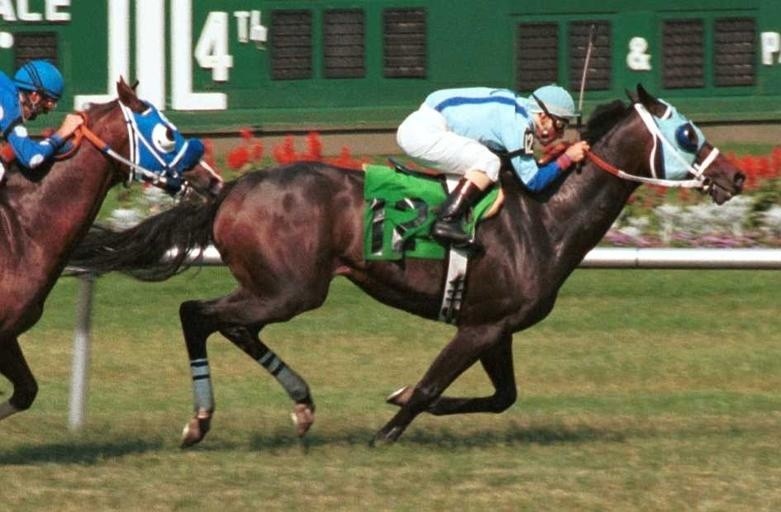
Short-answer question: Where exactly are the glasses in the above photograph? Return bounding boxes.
[23,61,56,113]
[534,96,569,133]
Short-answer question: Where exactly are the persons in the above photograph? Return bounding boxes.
[1,59,86,181]
[396,83,593,247]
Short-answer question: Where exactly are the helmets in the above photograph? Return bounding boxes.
[14,60,64,100]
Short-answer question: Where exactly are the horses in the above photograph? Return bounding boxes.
[58,83,746,449]
[0,75,223,421]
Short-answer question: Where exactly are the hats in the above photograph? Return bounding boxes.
[529,83,582,121]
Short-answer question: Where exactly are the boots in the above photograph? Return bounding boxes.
[430,176,484,254]
